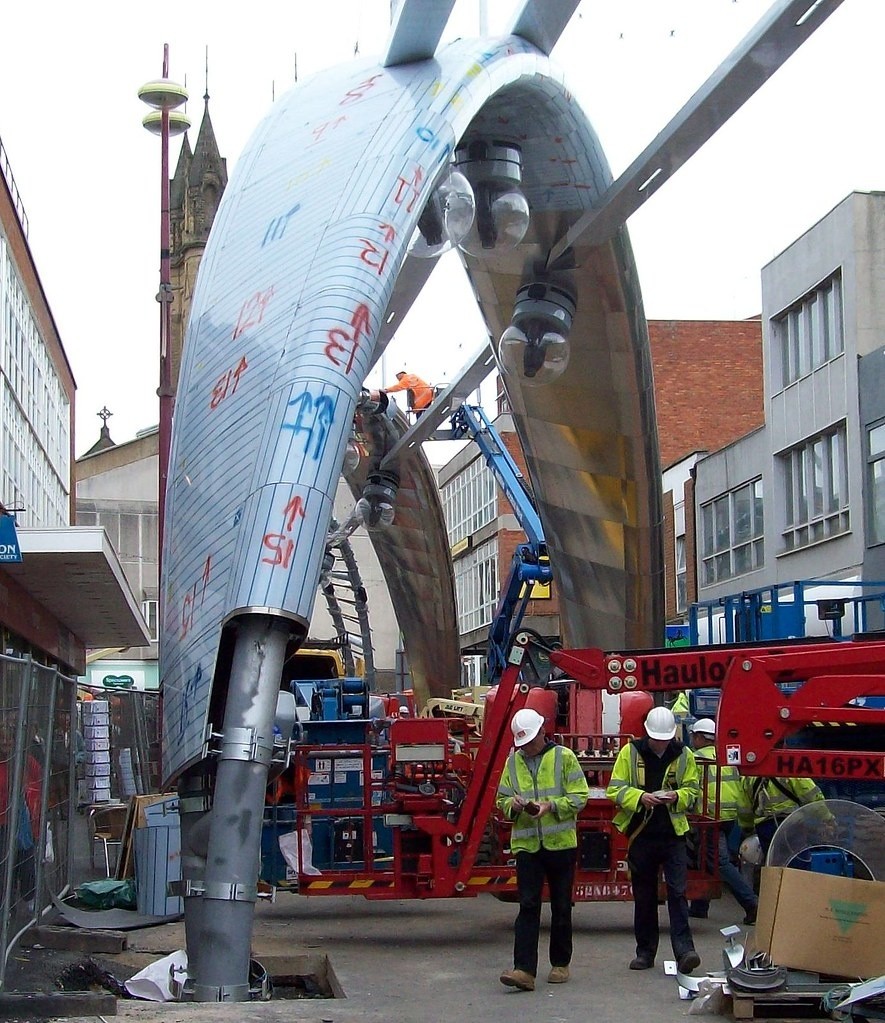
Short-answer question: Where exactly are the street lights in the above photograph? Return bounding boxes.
[139,41,195,596]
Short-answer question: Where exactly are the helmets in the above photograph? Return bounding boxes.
[509,708,543,747]
[644,706,678,740]
[739,834,764,865]
[691,718,716,739]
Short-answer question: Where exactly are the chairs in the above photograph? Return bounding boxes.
[87,806,129,879]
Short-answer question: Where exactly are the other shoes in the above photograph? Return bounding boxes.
[743,913,757,924]
[499,969,536,991]
[677,951,701,975]
[686,907,708,919]
[630,957,655,970]
[548,966,568,982]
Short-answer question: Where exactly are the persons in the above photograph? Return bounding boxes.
[495,708,590,990]
[384,371,434,420]
[736,776,839,895]
[685,717,759,925]
[607,706,701,974]
[670,689,689,742]
[0,713,84,931]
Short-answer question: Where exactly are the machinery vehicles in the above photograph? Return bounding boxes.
[262,382,885,900]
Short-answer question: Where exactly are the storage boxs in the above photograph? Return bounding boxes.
[112,748,136,796]
[83,701,111,803]
[755,866,885,980]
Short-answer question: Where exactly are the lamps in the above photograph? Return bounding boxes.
[317,552,335,591]
[445,134,531,259]
[340,422,360,475]
[354,471,399,533]
[405,148,474,260]
[498,265,578,387]
